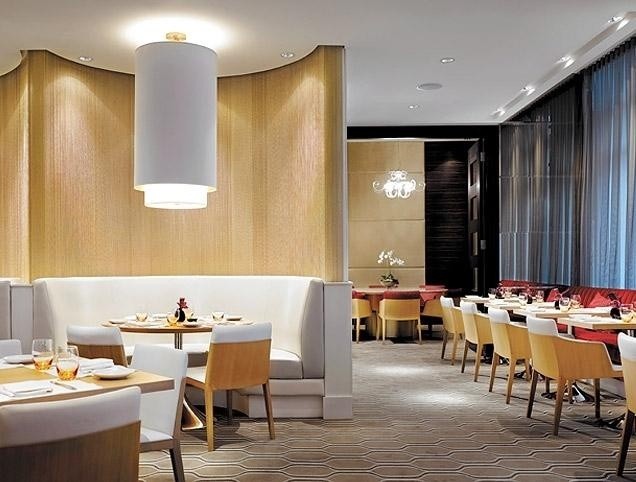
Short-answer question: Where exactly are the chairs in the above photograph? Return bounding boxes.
[1,385,142,482]
[186,321,276,452]
[0,339,22,359]
[129,342,189,482]
[65,324,129,367]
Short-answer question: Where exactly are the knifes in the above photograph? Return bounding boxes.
[50,381,77,391]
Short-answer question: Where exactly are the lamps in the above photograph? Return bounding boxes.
[132,32,217,209]
[370,169,426,199]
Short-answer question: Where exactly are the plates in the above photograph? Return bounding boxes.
[100,311,252,329]
[92,366,136,379]
[4,353,32,364]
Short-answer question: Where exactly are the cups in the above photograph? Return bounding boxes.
[32,339,53,369]
[54,346,79,379]
[488,283,635,325]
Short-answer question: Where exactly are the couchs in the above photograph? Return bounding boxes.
[29,275,323,420]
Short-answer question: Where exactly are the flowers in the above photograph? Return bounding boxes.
[377,248,405,280]
